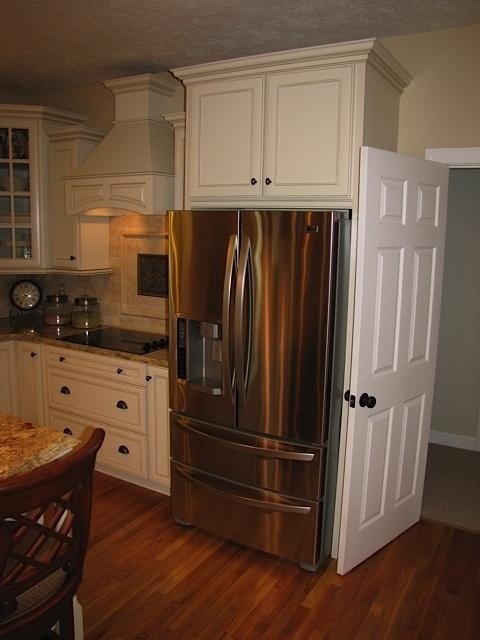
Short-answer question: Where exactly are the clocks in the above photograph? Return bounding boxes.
[10,279,43,310]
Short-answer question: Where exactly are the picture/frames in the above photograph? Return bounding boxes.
[136,253,168,298]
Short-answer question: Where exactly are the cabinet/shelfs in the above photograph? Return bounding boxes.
[0,103,115,275]
[0,340,172,498]
[167,38,416,211]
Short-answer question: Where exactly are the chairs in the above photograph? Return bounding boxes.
[0,426,106,640]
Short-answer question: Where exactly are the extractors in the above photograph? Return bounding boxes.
[60,73,174,216]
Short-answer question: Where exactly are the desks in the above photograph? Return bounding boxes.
[0,408,83,485]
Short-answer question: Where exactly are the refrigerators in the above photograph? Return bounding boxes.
[167,208,352,575]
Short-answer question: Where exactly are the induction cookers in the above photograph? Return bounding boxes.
[56,327,169,355]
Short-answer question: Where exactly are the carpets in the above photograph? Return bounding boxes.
[420,442,480,535]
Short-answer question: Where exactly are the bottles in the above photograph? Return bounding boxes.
[42,294,71,327]
[71,296,100,329]
